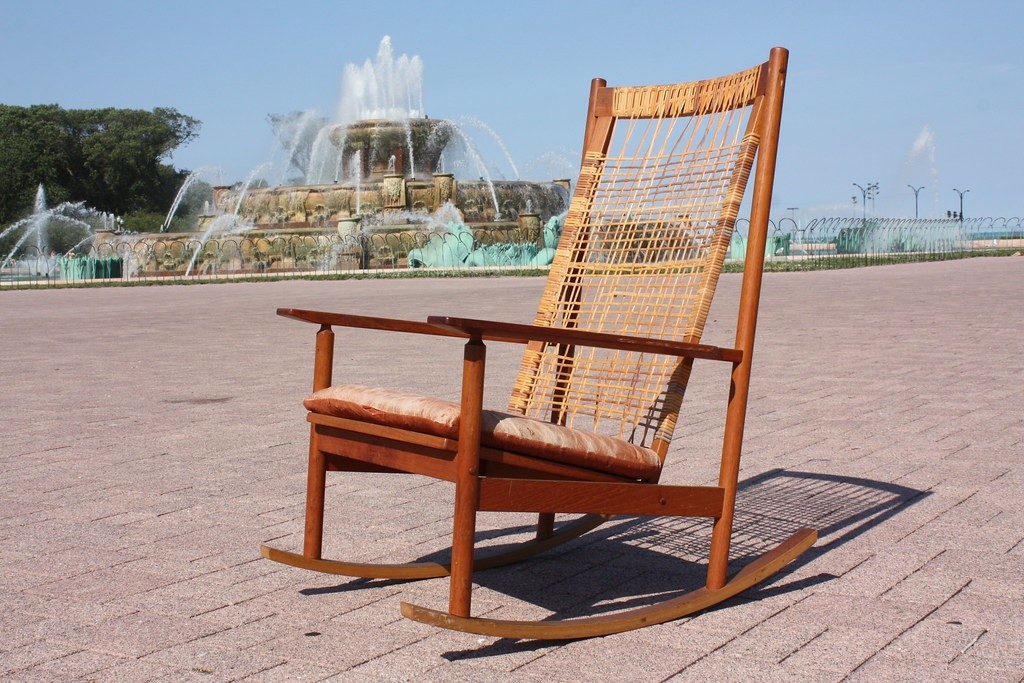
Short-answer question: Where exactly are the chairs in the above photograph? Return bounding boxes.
[259,45,820,646]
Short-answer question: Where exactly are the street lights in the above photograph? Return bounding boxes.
[953,188,970,222]
[852,183,877,220]
[908,185,925,219]
[786,207,800,244]
